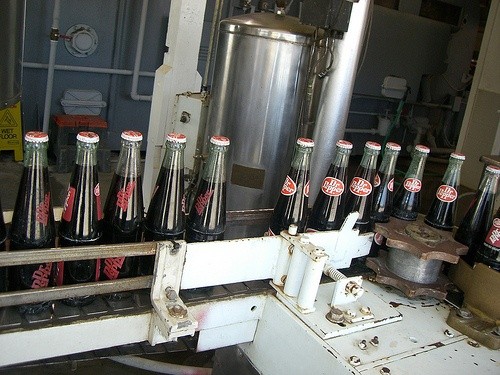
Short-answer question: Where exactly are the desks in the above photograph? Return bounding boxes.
[50,112,107,173]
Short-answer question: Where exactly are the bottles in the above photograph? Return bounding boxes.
[343,140,381,234]
[455,164,500,272]
[390,144,430,222]
[99,129,144,301]
[54,131,104,307]
[268,137,315,236]
[306,139,353,232]
[370,142,402,232]
[137,133,190,295]
[0,201,10,295]
[424,152,466,231]
[184,135,230,293]
[10,131,56,314]
